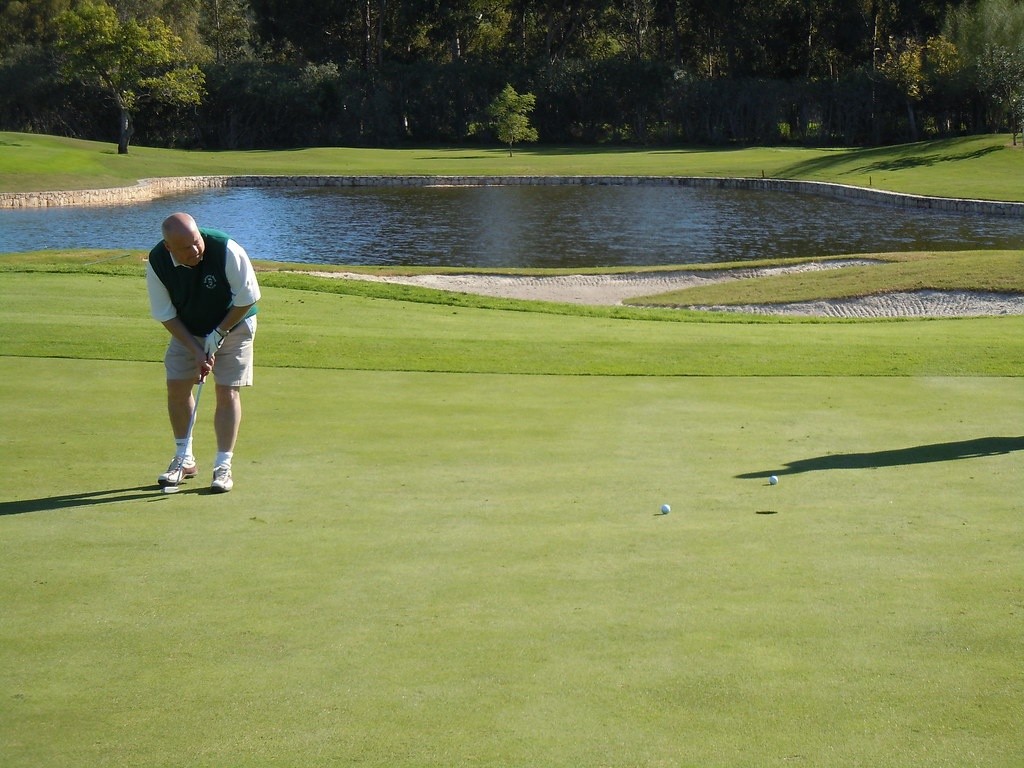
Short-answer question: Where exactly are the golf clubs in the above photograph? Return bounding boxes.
[161,333,209,495]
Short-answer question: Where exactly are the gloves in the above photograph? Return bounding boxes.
[204,327,230,363]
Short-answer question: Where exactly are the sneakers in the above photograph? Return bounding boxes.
[158,457,197,486]
[210,463,234,493]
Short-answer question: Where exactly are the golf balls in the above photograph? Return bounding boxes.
[769,475,778,485]
[660,504,671,514]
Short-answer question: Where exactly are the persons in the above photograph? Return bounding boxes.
[146,212,262,492]
